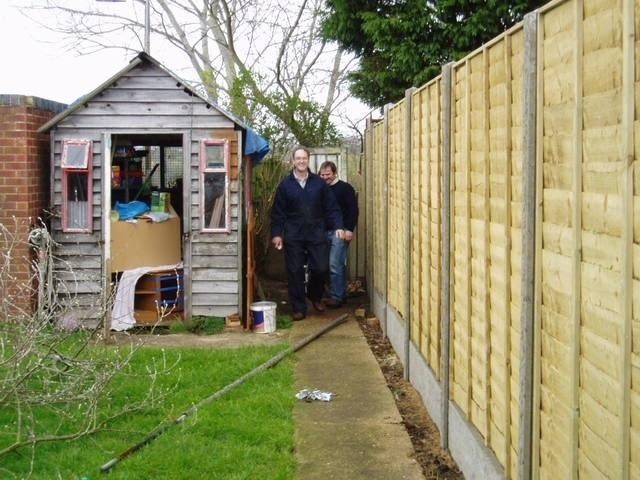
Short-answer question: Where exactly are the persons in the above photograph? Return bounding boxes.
[319,161,358,308]
[271,146,345,321]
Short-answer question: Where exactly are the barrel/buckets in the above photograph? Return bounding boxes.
[250,301,278,334]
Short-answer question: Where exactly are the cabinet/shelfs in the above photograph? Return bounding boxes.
[110,268,183,326]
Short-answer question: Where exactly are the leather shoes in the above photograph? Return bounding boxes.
[325,300,342,307]
[312,300,325,312]
[293,312,305,321]
